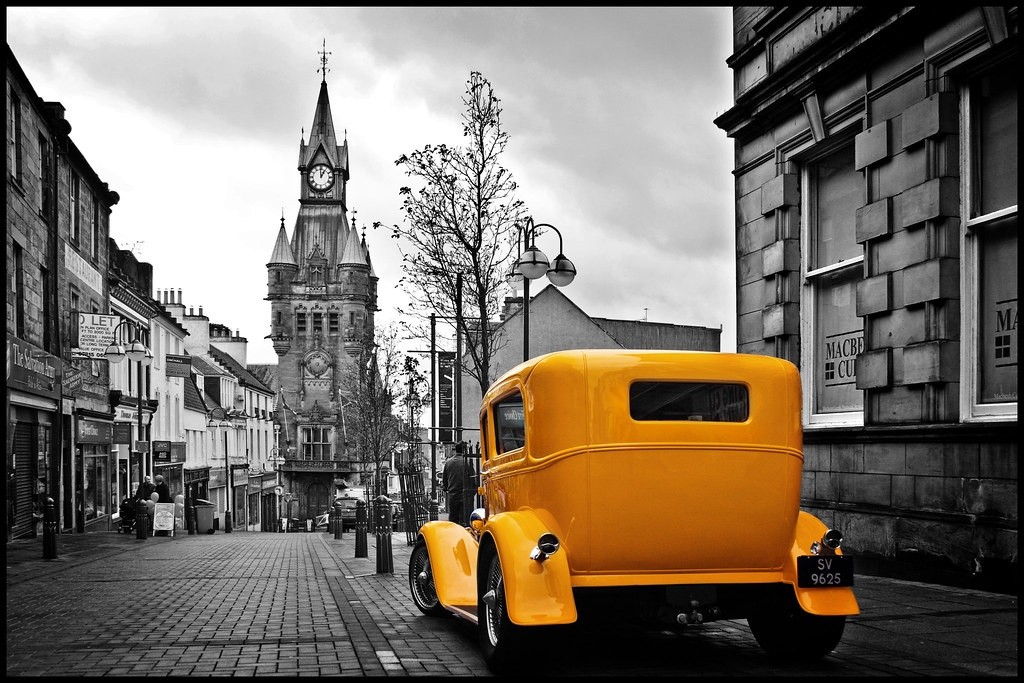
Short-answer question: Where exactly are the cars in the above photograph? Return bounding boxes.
[409,347,862,675]
[333,496,360,531]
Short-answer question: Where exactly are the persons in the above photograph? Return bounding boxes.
[154,475,170,536]
[136,475,156,501]
[443,441,477,526]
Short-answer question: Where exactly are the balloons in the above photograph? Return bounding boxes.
[174,495,185,517]
[147,492,159,515]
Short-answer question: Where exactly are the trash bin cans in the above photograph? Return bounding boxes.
[193,498,215,535]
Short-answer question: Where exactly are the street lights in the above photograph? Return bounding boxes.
[267,447,286,533]
[503,218,577,363]
[206,408,234,534]
[103,320,153,492]
